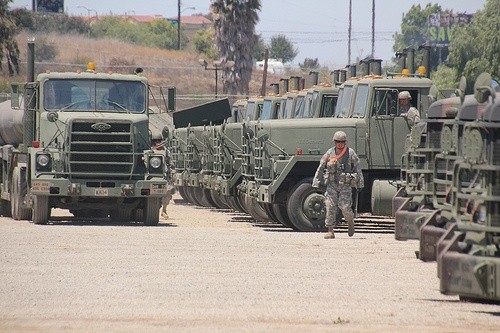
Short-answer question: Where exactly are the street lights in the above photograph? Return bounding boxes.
[198,58,236,99]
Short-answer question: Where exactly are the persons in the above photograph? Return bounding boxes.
[399,91,420,131]
[150,132,177,220]
[312,131,365,239]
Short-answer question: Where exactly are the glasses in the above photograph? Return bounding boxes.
[335,140,345,143]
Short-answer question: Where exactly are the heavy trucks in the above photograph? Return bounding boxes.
[169,42,500,304]
[0,40,177,225]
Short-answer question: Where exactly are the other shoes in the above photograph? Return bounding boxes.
[323,230,335,239]
[160,211,169,218]
[348,221,355,237]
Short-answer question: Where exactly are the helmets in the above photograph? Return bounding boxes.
[398,91,412,100]
[333,131,347,142]
